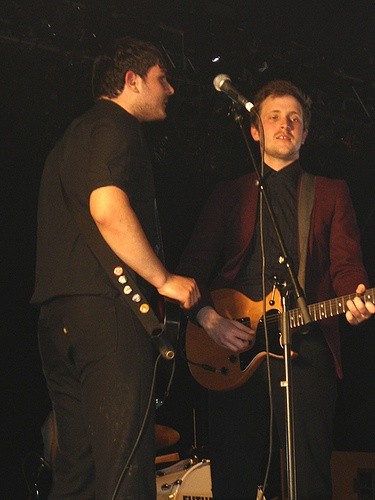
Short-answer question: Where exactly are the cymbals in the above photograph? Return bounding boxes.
[45,413,180,450]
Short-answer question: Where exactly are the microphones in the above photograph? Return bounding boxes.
[213,73,255,112]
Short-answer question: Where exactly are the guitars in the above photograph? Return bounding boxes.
[150,301,182,396]
[185,286,375,392]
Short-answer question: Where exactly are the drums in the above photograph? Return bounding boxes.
[157,460,265,500]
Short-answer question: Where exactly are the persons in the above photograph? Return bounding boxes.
[174,79,375,500]
[28,36,202,500]
